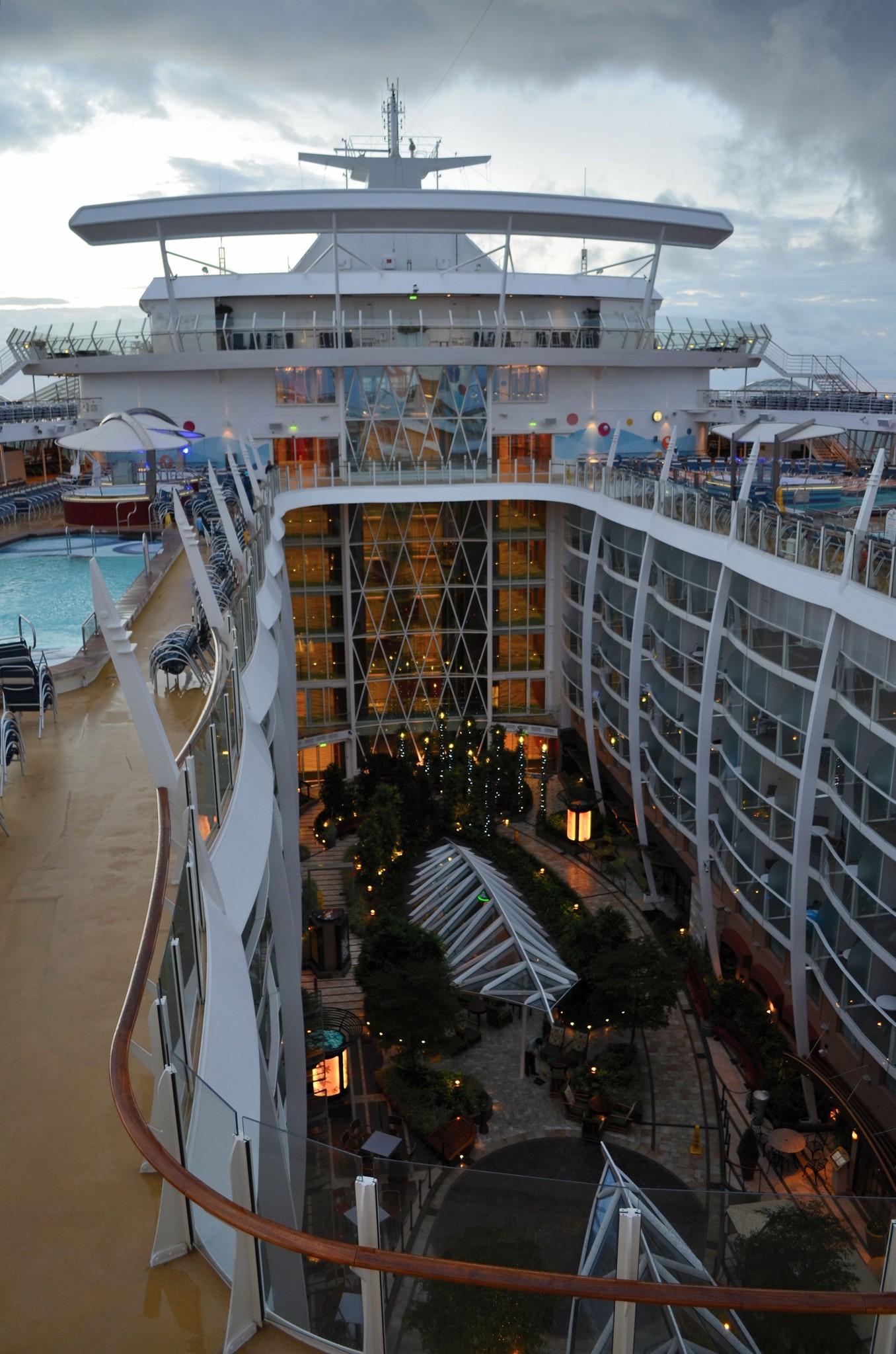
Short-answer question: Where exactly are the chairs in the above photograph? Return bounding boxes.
[339,1114,421,1179]
[750,1116,774,1159]
[801,1125,828,1161]
[537,1023,566,1064]
[485,1004,514,1029]
[766,1148,791,1181]
[0,636,60,839]
[148,470,251,693]
[802,1157,828,1186]
[560,1026,590,1070]
[580,1108,607,1146]
[577,454,895,582]
[604,1098,642,1136]
[0,472,87,525]
[559,1083,593,1123]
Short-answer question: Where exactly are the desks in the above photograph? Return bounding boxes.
[587,1093,614,1119]
[545,1053,573,1087]
[467,1000,490,1027]
[766,1128,807,1172]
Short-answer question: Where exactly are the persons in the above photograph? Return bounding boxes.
[524,1037,544,1076]
[196,460,276,544]
